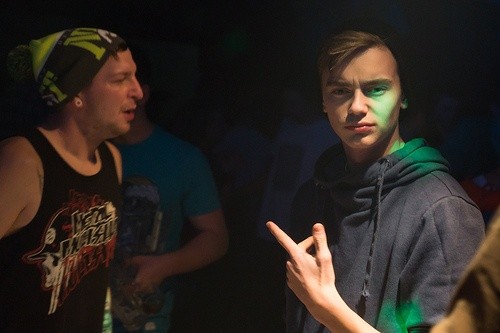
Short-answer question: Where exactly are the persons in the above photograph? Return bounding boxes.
[0,26,145,333]
[264,21,486,333]
[106,72,232,333]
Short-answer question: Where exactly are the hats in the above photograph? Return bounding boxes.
[9,27,126,111]
